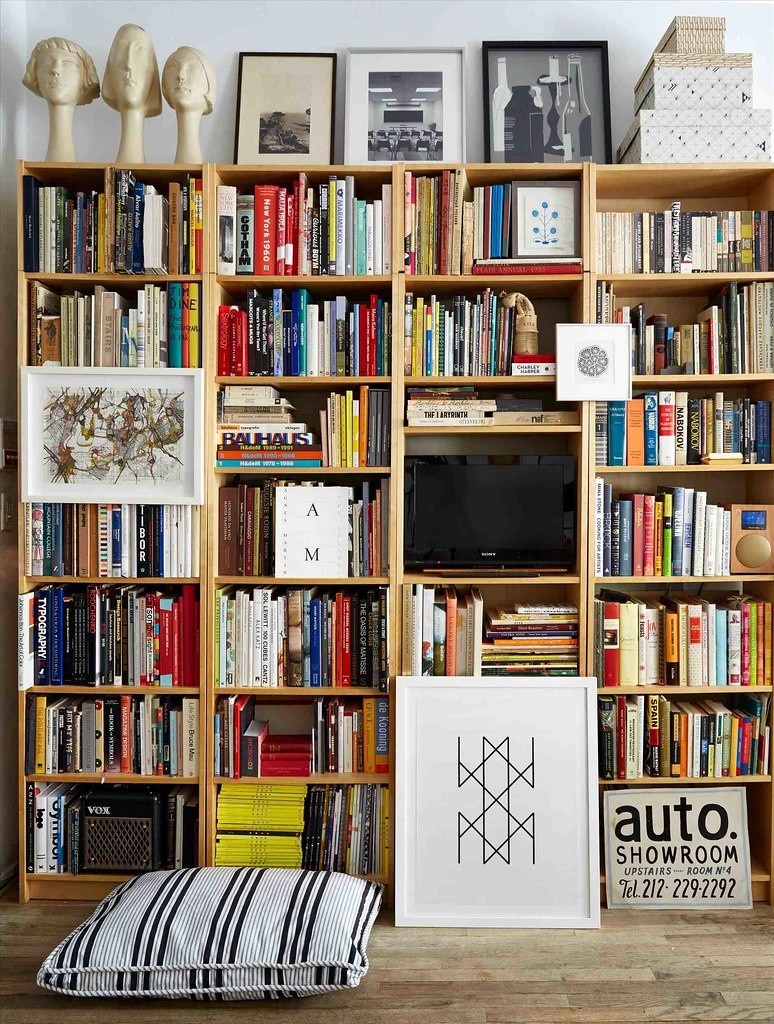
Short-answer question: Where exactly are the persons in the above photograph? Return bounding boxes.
[22,24,101,162]
[161,46,216,164]
[101,23,163,164]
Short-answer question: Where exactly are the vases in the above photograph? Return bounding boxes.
[482,40,613,165]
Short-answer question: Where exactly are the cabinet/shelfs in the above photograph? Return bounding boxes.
[16,158,774,906]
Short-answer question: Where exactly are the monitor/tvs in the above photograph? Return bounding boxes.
[403,455,578,570]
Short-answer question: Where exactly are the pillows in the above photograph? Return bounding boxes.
[35,865,385,1001]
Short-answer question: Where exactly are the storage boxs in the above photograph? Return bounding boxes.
[613,110,774,162]
[653,16,726,53]
[633,52,753,116]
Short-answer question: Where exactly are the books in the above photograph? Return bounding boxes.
[24,167,771,880]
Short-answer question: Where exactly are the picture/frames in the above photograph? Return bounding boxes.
[394,675,603,931]
[601,785,755,911]
[511,181,582,259]
[340,44,469,165]
[556,322,633,402]
[19,365,208,507]
[233,51,337,165]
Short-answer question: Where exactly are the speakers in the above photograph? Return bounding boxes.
[77,787,165,875]
[731,504,774,574]
